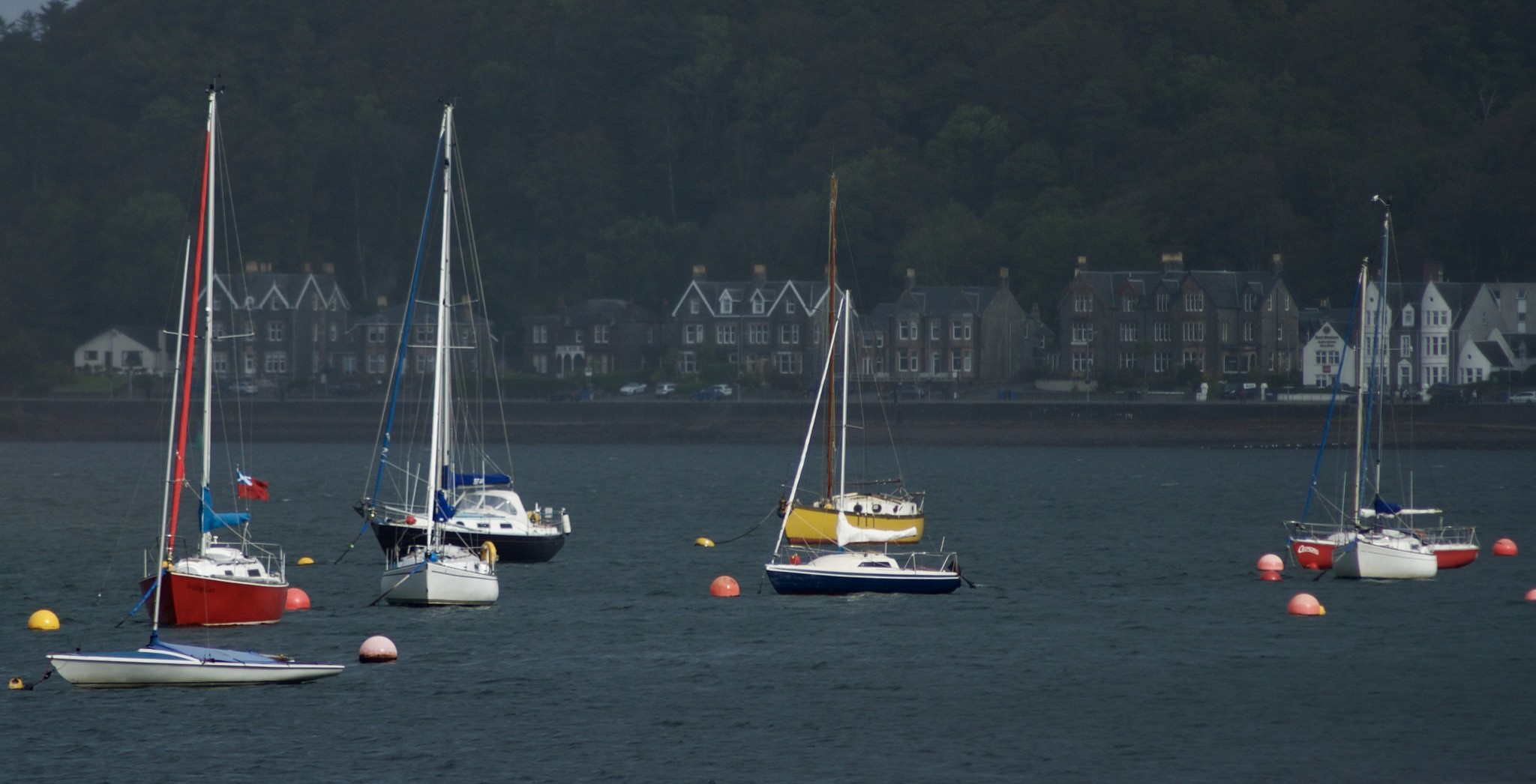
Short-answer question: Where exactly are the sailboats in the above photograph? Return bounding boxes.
[1286,202,1482,571]
[138,89,285,628]
[380,190,503,608]
[350,107,575,565]
[1331,254,1440,582]
[48,234,345,687]
[762,290,962,594]
[782,171,927,545]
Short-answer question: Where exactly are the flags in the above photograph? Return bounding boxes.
[1375,492,1403,514]
[236,467,270,503]
